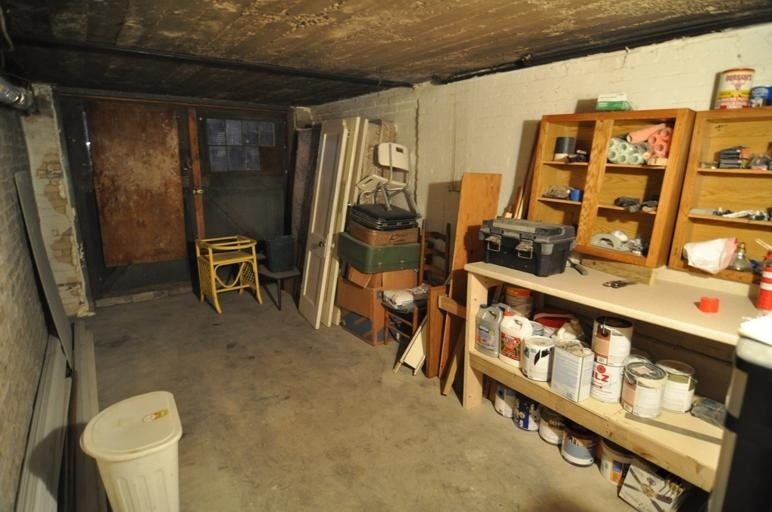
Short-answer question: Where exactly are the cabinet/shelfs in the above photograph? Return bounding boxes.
[461,260,772,492]
[528,107,696,268]
[667,105,772,284]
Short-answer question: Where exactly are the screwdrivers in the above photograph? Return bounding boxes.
[567,259,588,276]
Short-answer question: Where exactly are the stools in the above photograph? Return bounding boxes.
[258,235,300,311]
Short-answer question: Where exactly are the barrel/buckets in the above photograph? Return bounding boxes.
[714,69,756,110]
[473,284,697,421]
[491,379,633,485]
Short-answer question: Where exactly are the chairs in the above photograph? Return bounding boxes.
[384,220,451,346]
[356,142,416,213]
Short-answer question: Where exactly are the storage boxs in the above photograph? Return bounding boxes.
[334,204,420,347]
